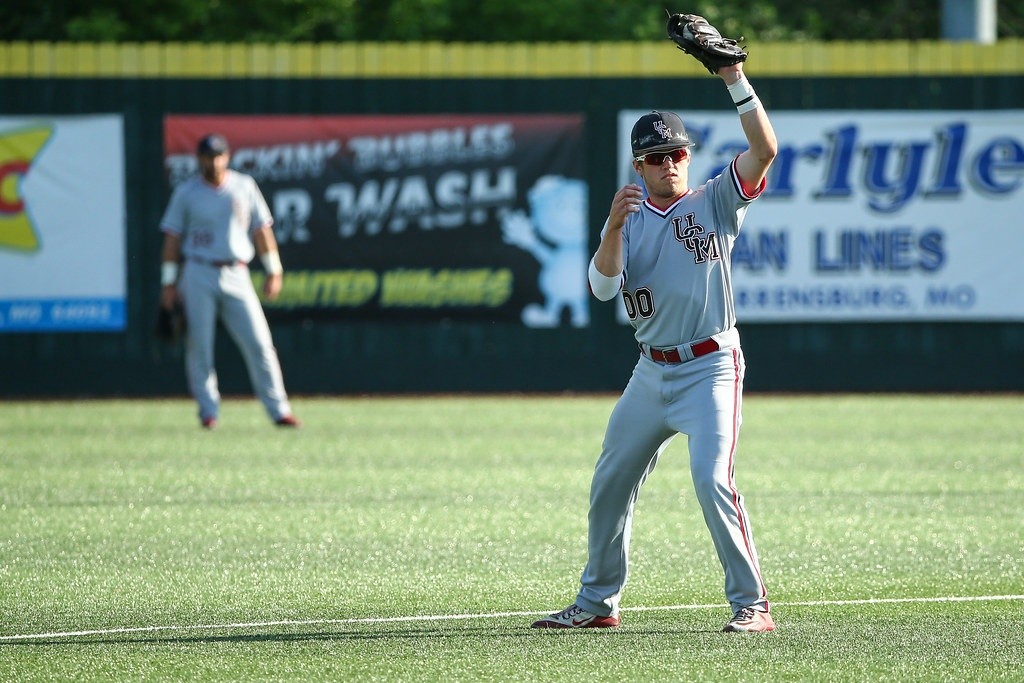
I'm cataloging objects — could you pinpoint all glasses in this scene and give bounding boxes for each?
[640,148,687,165]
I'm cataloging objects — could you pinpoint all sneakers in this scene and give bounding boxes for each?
[532,604,620,629]
[722,606,775,632]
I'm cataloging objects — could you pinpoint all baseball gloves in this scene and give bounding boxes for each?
[668,13,747,75]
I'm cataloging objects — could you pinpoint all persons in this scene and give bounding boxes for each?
[530,8,778,632]
[160,134,301,429]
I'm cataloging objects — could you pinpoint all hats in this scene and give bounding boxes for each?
[631,110,695,157]
[198,133,226,156]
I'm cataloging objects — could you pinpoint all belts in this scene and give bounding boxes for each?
[641,340,719,365]
[191,260,244,268]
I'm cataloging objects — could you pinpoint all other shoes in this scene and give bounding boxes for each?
[203,419,216,431]
[276,415,300,426]
